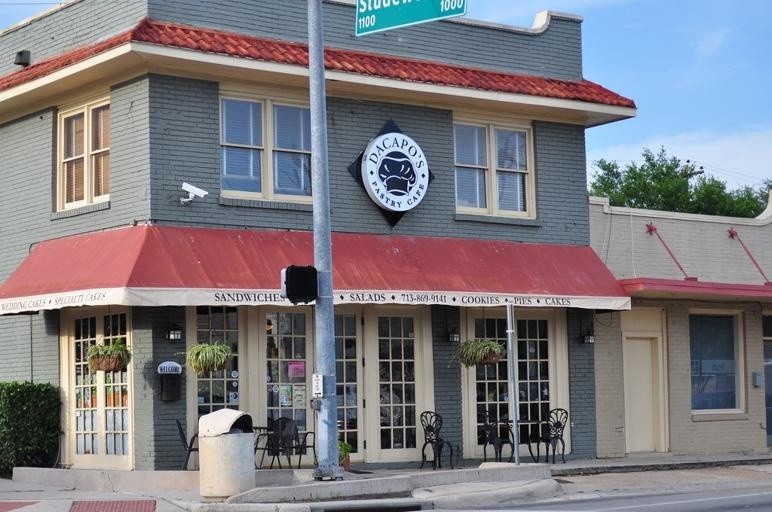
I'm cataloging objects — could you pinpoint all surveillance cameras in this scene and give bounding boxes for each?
[181,181,209,199]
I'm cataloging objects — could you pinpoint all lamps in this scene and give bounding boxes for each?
[165,320,182,341]
[584,328,594,344]
[448,328,459,342]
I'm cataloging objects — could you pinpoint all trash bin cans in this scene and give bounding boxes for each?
[199,409,256,498]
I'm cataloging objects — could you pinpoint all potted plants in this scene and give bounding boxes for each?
[86,338,131,372]
[338,439,352,471]
[454,338,505,368]
[187,342,232,374]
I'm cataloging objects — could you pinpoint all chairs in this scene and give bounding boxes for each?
[420,411,454,470]
[484,410,515,462]
[534,408,568,463]
[175,417,319,471]
